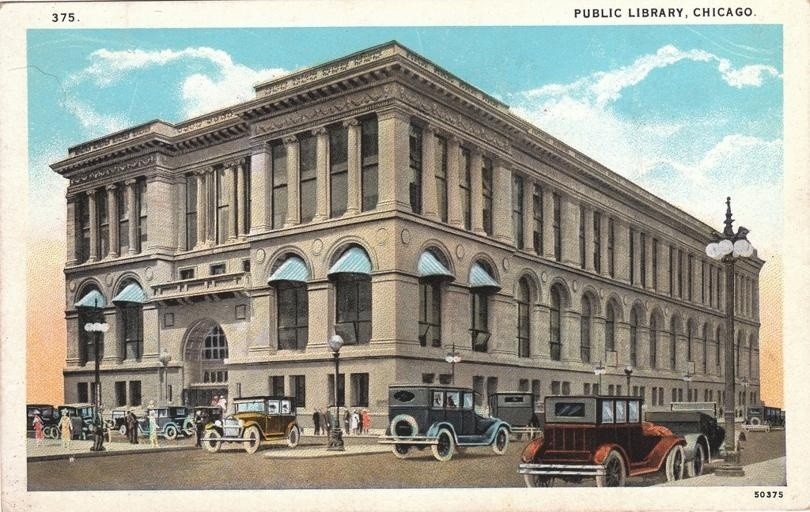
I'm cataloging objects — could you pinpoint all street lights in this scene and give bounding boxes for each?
[702,196,754,464]
[624,365,634,396]
[83,298,110,414]
[327,334,345,427]
[444,341,462,384]
[592,359,607,394]
[158,350,172,401]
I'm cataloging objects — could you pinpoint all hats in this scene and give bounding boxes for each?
[61,408,69,413]
[33,410,40,414]
[362,409,369,412]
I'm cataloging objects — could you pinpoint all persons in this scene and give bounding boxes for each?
[218,395,227,411]
[149,409,160,447]
[123,410,139,444]
[90,405,106,450]
[56,408,73,452]
[210,395,219,406]
[311,404,370,434]
[33,410,45,448]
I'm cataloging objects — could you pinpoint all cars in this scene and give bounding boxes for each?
[511,391,725,487]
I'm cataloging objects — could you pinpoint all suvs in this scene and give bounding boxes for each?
[376,382,545,462]
[27,394,302,454]
[745,405,785,434]
